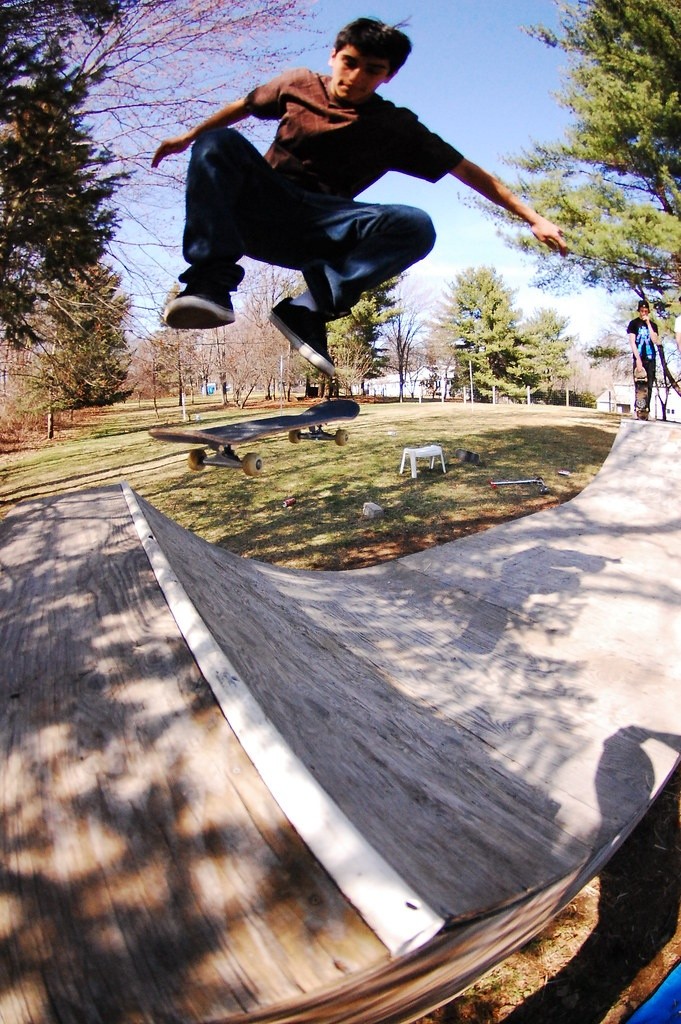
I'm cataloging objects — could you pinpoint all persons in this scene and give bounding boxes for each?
[674,295,681,352]
[151,18,571,376]
[627,300,659,419]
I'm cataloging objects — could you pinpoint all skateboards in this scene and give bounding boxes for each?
[454,448,480,464]
[148,399,361,476]
[634,367,651,419]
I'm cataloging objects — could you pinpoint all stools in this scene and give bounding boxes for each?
[400,445,446,479]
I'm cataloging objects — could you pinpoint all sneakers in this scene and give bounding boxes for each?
[164,283,235,329]
[269,297,336,377]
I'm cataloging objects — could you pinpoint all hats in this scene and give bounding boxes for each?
[637,301,649,311]
